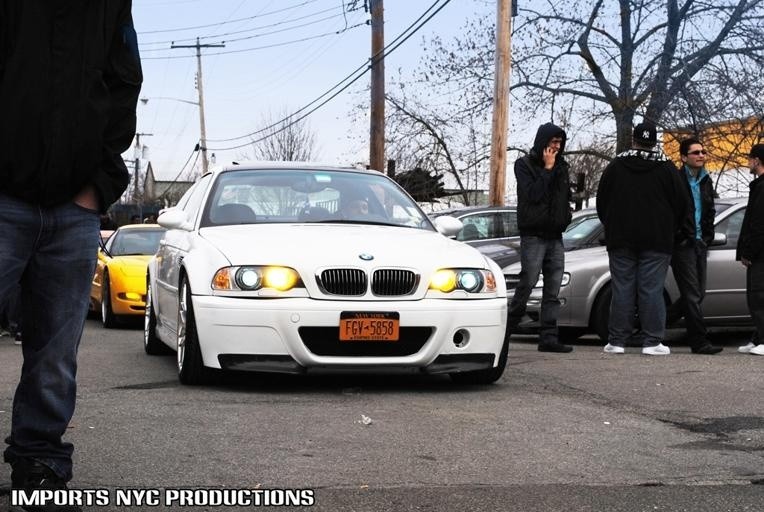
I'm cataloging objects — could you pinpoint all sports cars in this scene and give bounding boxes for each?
[88,223,171,329]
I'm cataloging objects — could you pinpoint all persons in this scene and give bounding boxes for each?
[595,122,684,355]
[463,224,479,240]
[668,138,725,355]
[0,1,140,510]
[506,122,578,354]
[336,188,372,221]
[735,144,763,354]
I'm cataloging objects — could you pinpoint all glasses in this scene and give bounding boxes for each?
[688,150,707,156]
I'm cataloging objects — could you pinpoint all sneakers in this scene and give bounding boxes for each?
[10,460,83,512]
[603,343,625,354]
[642,342,671,355]
[738,342,764,355]
[538,345,573,353]
[691,345,723,354]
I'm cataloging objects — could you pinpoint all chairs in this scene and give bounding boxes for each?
[214,204,257,225]
[299,206,329,220]
[456,224,478,240]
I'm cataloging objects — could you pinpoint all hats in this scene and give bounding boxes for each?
[634,123,657,146]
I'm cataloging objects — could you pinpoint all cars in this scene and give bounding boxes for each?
[140,160,513,392]
[421,205,606,270]
[501,196,759,349]
[98,230,115,251]
